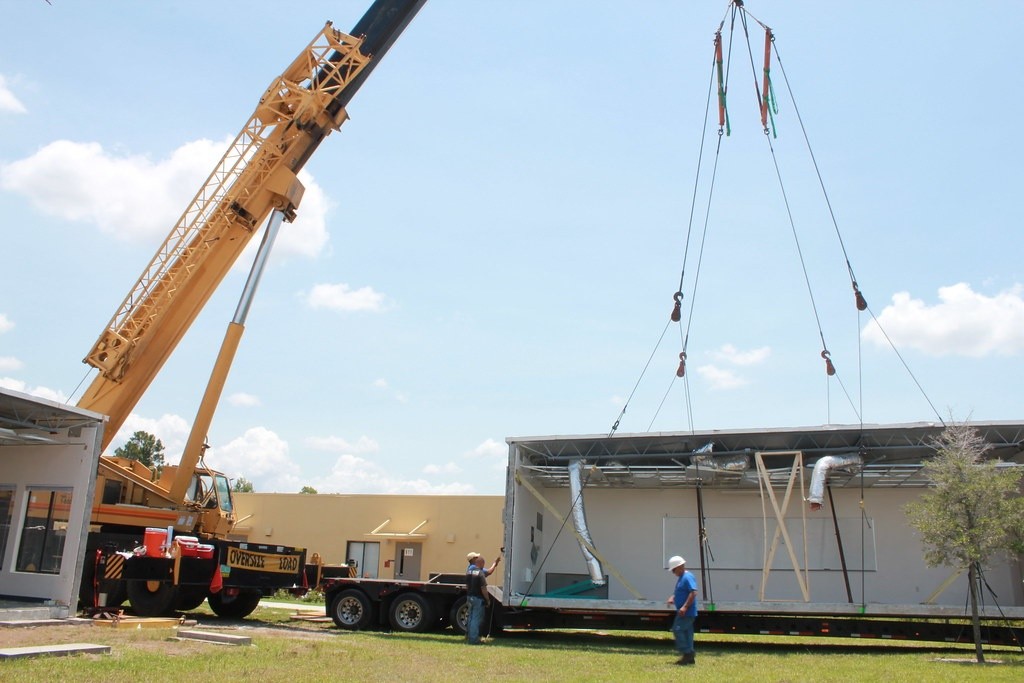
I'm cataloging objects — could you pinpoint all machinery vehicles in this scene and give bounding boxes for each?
[1,0,427,621]
[320,530,495,634]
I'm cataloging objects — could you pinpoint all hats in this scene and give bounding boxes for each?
[467,552,480,561]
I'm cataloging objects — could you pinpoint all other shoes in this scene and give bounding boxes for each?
[475,641,485,645]
[675,651,695,664]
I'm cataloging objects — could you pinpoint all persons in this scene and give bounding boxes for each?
[466,552,502,645]
[667,556,698,665]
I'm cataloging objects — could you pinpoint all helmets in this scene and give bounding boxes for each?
[669,555,686,571]
